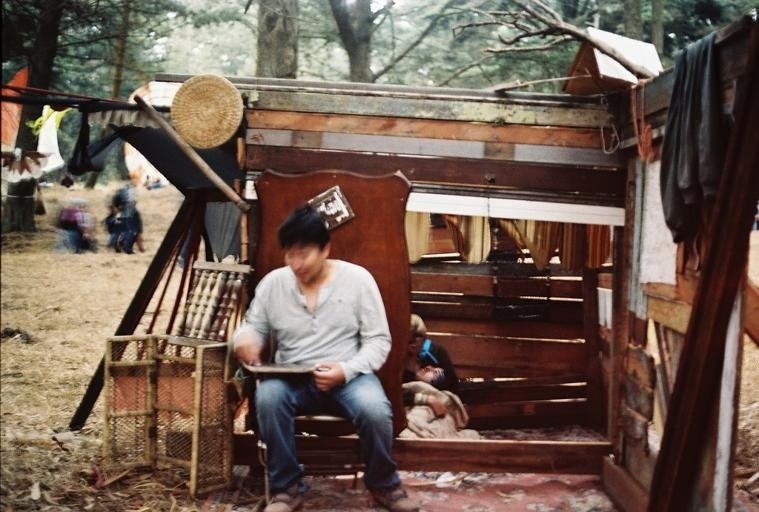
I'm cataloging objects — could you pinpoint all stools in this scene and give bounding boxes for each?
[248,410,363,492]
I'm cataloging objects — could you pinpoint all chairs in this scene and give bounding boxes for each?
[103,254,253,498]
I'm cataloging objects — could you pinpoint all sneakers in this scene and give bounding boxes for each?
[263,482,303,512]
[373,486,419,512]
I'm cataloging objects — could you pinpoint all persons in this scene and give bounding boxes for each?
[54,176,145,256]
[402,315,484,440]
[230,202,418,511]
